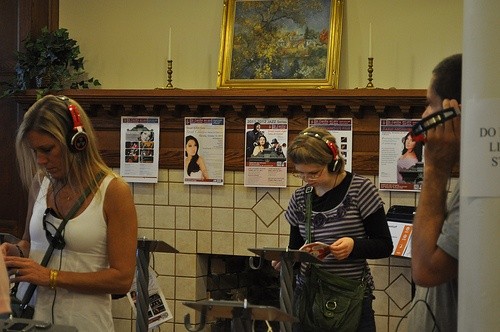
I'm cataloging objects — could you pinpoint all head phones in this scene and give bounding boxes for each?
[302,132,344,174]
[55,95,88,153]
[410,106,461,142]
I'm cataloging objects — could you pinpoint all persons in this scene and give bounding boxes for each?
[272,126,394,332]
[1,94,138,332]
[412,54,462,332]
[397,132,423,184]
[184,136,209,179]
[247,122,285,162]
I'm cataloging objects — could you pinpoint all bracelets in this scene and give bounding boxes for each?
[49,268,58,291]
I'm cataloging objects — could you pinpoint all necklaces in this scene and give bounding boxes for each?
[63,188,70,201]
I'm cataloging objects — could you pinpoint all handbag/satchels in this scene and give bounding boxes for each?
[293,263,368,332]
[8,290,36,332]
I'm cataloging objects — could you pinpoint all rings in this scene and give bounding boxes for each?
[15,269,21,275]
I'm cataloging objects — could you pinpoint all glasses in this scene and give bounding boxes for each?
[292,164,325,181]
